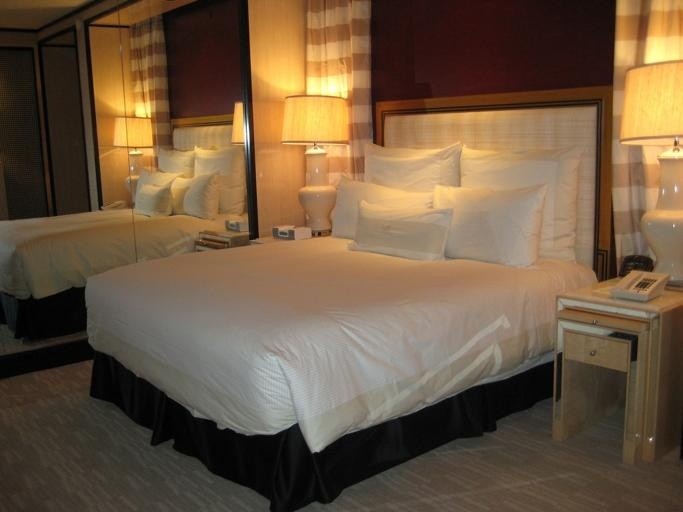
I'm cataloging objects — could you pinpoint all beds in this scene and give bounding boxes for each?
[81,81,616,511]
[0,113,248,348]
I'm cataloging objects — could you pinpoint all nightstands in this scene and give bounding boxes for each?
[251,235,326,247]
[191,232,248,252]
[551,276,683,468]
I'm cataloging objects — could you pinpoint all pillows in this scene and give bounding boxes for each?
[435,180,549,272]
[167,168,221,223]
[346,199,454,264]
[459,142,586,265]
[361,142,459,194]
[134,169,183,212]
[132,182,174,219]
[193,146,247,217]
[156,145,197,177]
[329,173,434,243]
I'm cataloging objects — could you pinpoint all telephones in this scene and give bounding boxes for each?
[609,270,670,302]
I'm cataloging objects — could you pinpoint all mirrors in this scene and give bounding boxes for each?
[0,0,261,380]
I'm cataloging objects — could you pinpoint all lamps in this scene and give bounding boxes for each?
[113,117,155,206]
[230,100,249,217]
[279,95,353,240]
[619,59,683,287]
